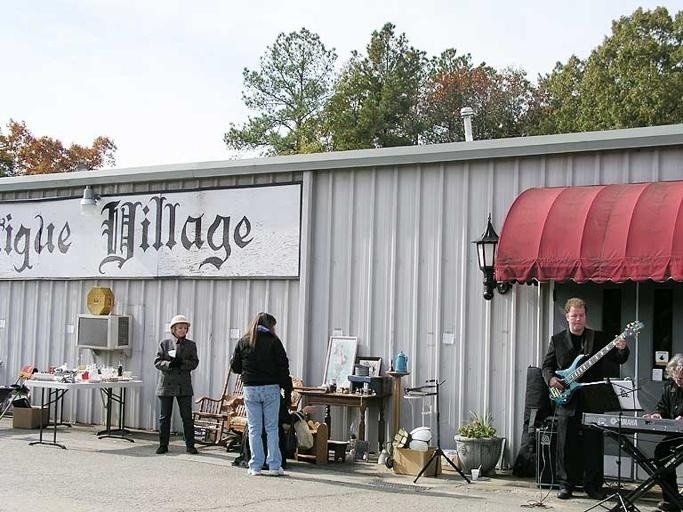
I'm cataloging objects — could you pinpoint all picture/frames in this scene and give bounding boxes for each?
[355,356,382,377]
[321,335,358,393]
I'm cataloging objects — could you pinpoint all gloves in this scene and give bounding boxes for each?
[169,360,182,369]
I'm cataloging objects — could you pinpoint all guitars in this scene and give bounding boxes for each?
[549,320,645,405]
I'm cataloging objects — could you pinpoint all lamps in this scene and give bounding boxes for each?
[76,183,102,219]
[468,210,538,301]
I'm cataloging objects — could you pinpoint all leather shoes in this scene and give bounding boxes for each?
[155,444,168,455]
[556,487,573,500]
[585,489,607,500]
[656,500,682,511]
[186,445,199,454]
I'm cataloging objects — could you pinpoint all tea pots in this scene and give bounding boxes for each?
[394,350,409,371]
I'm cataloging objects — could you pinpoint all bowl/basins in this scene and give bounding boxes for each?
[355,366,369,376]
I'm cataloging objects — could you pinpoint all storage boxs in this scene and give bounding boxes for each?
[11,406,48,430]
[392,444,442,477]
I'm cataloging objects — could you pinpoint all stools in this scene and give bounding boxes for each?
[326,439,350,465]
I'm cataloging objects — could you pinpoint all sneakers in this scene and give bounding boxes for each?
[247,466,262,477]
[269,467,285,476]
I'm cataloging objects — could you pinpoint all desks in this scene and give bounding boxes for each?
[299,389,392,442]
[22,378,143,451]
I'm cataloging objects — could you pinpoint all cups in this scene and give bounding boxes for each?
[470,469,479,482]
[49,362,139,382]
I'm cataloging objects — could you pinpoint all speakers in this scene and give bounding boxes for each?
[534,427,605,489]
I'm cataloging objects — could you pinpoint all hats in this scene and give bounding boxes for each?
[169,314,190,330]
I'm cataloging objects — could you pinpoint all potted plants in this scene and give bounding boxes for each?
[452,406,504,476]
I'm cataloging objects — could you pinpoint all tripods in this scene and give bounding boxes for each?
[585,411,649,511]
[413,379,472,486]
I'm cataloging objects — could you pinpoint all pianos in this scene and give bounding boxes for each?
[581,411,683,432]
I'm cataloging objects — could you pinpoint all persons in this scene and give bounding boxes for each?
[155,314,199,454]
[231,312,289,476]
[540,297,631,500]
[232,373,293,468]
[645,354,683,511]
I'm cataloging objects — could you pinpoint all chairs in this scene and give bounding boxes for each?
[0,368,38,422]
[191,362,243,447]
[223,373,304,453]
